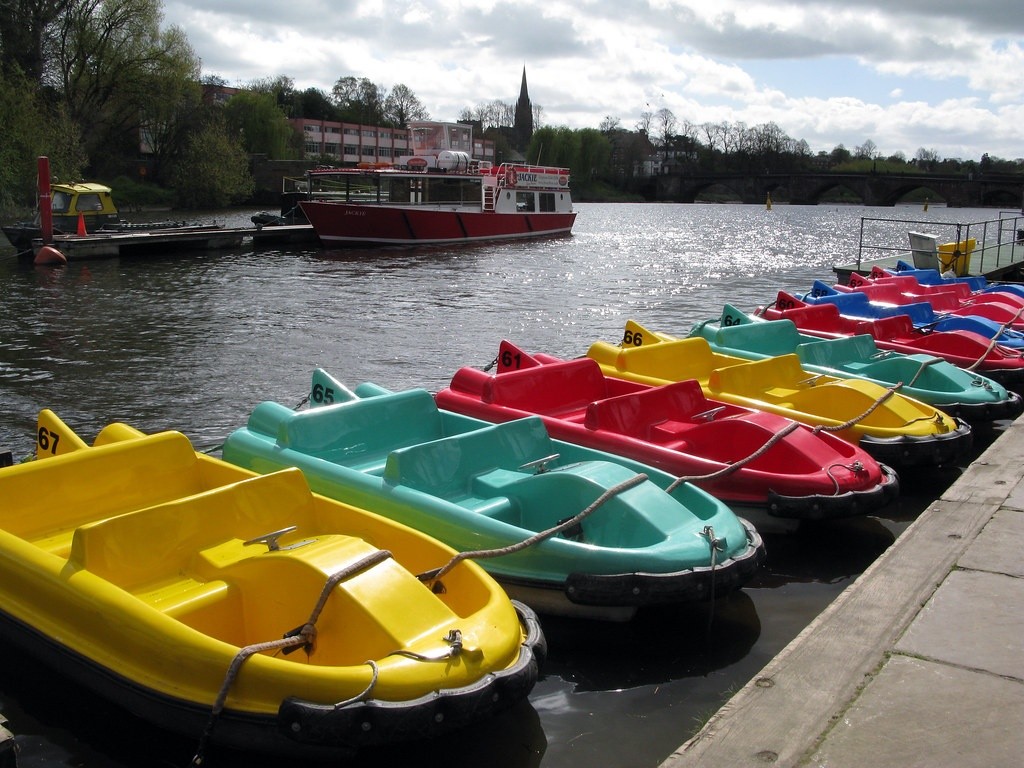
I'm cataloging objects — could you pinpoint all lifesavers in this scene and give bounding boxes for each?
[506,166,518,184]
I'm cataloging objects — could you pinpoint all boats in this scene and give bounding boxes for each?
[687,304,1024,422]
[223,368,767,622]
[1,176,225,249]
[435,339,900,525]
[0,409,549,758]
[297,150,577,249]
[586,321,973,466]
[754,260,1024,372]
